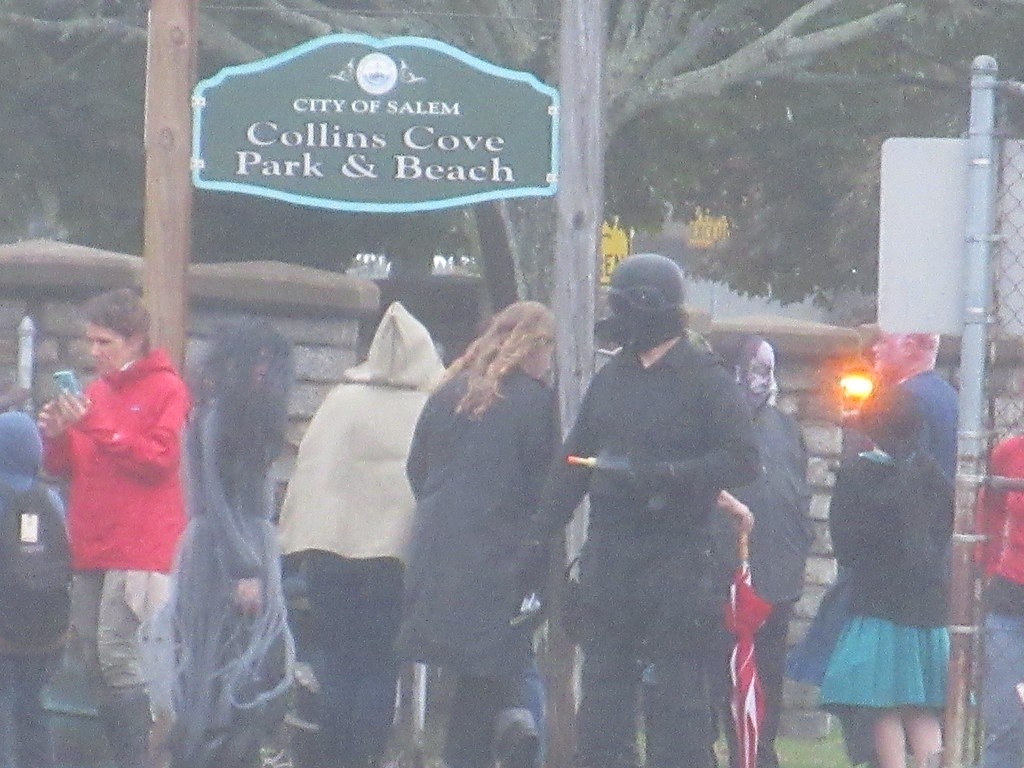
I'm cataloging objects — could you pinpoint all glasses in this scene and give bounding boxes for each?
[608,287,687,318]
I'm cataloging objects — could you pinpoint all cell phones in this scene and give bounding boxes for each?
[53,372,78,402]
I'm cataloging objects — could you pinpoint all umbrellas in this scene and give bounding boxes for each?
[721,519,776,768]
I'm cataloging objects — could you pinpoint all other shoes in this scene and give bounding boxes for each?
[496,710,541,768]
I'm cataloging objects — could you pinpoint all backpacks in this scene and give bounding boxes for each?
[1,478,73,643]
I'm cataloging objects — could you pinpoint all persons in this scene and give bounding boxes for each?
[0,411,73,768]
[136,320,295,768]
[403,301,564,768]
[272,300,447,768]
[694,336,810,768]
[780,325,959,768]
[817,386,958,768]
[970,430,1023,768]
[494,253,760,768]
[36,289,193,768]
